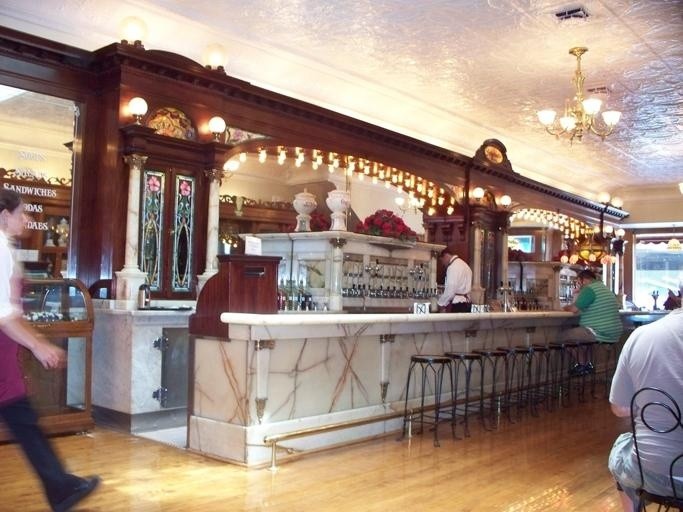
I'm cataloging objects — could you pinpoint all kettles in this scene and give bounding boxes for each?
[137,284,151,311]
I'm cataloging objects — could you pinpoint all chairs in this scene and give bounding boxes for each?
[617,386,683,511]
[396,341,614,446]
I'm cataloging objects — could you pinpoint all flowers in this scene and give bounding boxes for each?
[356,208,415,241]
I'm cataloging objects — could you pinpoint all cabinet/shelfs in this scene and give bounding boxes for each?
[22,204,71,279]
[22,278,96,435]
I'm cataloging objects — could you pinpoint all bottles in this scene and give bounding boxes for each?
[277,279,312,311]
[512,290,527,312]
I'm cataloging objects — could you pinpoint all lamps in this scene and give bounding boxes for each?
[560,191,625,264]
[665,225,680,250]
[500,195,510,209]
[535,45,621,143]
[127,97,147,124]
[207,116,227,143]
[474,186,484,204]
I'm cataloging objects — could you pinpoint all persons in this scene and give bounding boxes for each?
[437,248,472,311]
[554,269,623,377]
[666,283,682,307]
[0,188,99,512]
[608,279,682,511]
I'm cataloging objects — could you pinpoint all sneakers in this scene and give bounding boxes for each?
[567,363,593,375]
[52,475,98,511]
